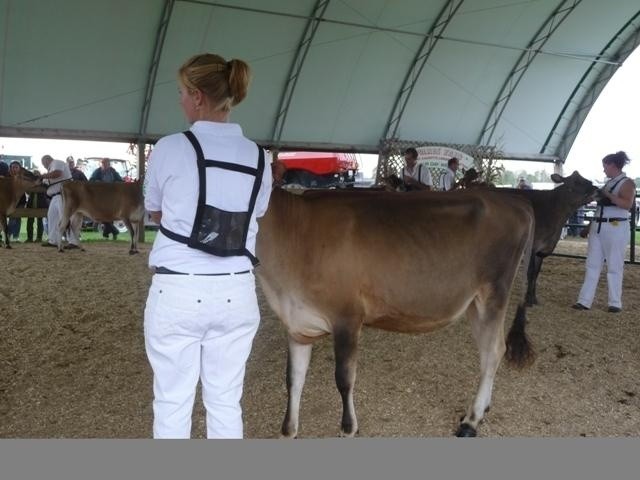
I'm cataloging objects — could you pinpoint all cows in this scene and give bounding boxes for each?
[496,170,601,308]
[56,174,145,255]
[0,165,48,250]
[252,184,536,439]
[272,166,484,191]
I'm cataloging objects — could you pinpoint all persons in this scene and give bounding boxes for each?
[142,53,274,440]
[0,155,124,250]
[438,157,460,191]
[570,151,636,312]
[515,177,534,188]
[396,147,433,192]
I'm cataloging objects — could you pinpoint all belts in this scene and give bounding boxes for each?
[51,190,61,198]
[595,218,627,222]
[155,266,250,275]
